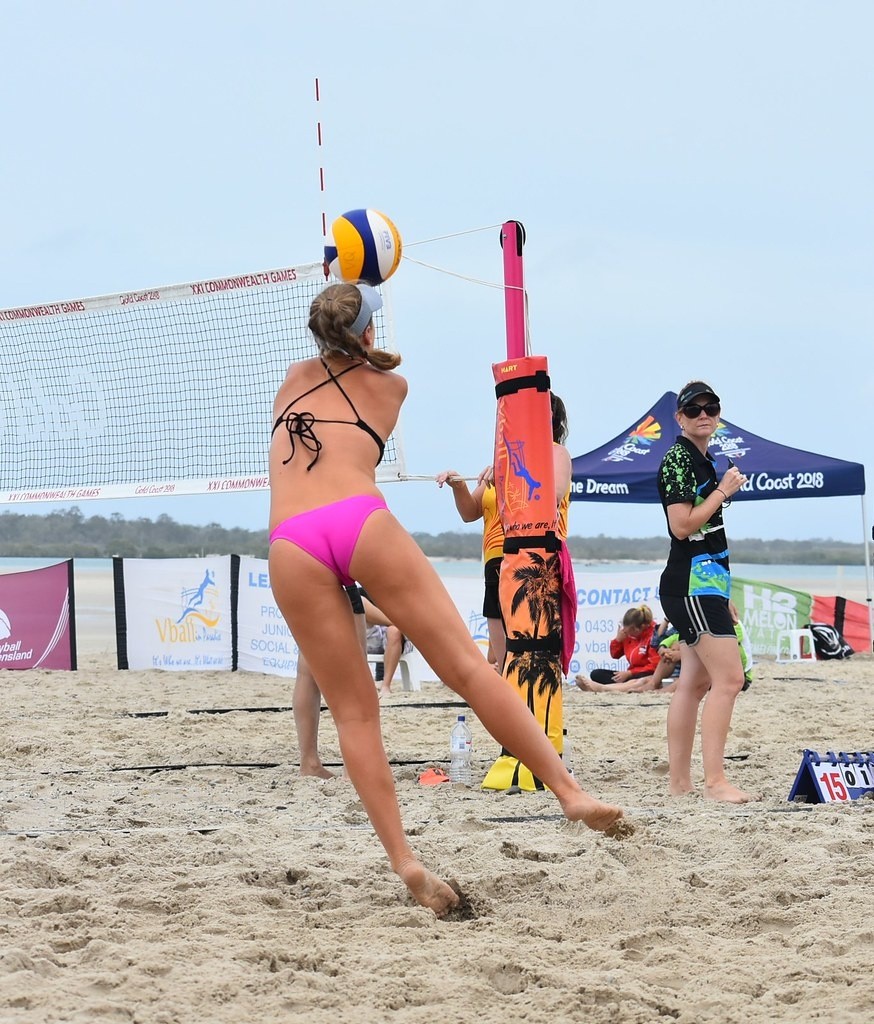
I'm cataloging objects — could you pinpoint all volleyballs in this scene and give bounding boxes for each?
[322,207,403,287]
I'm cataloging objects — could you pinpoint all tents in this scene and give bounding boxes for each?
[569,392,874,655]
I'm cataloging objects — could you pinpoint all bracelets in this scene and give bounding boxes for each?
[713,486,729,501]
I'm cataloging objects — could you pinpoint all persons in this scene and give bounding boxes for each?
[267,281,625,918]
[436,390,579,678]
[574,381,764,806]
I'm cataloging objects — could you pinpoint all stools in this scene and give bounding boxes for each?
[776,628,817,665]
[365,653,421,691]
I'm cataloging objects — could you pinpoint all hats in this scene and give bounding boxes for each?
[347,284,383,341]
[677,383,720,407]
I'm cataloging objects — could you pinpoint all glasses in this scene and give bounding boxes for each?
[682,403,720,419]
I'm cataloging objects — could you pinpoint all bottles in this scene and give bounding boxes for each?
[449,715,472,784]
